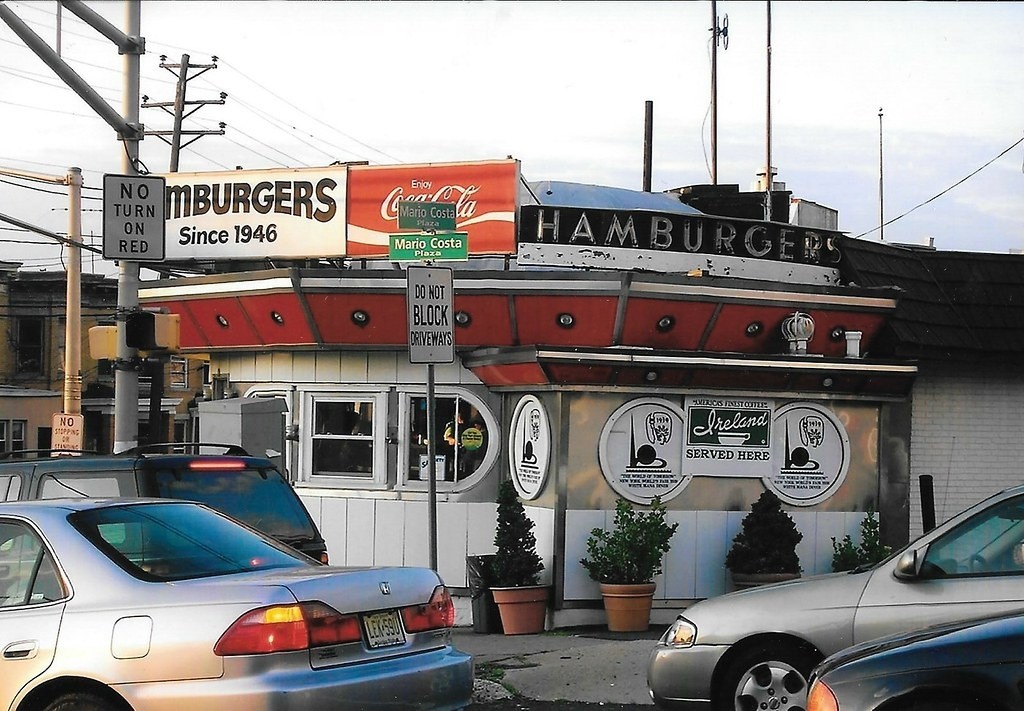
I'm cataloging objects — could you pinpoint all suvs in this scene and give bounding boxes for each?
[0,442,330,574]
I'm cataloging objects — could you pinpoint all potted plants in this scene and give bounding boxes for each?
[724,489,804,589]
[579,495,679,631]
[488,480,551,635]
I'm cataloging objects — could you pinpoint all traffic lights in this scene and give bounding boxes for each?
[125,311,180,355]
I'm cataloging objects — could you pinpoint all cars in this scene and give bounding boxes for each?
[645,483,1024,711]
[0,498,476,711]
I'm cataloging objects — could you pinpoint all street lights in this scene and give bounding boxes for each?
[877,107,885,242]
